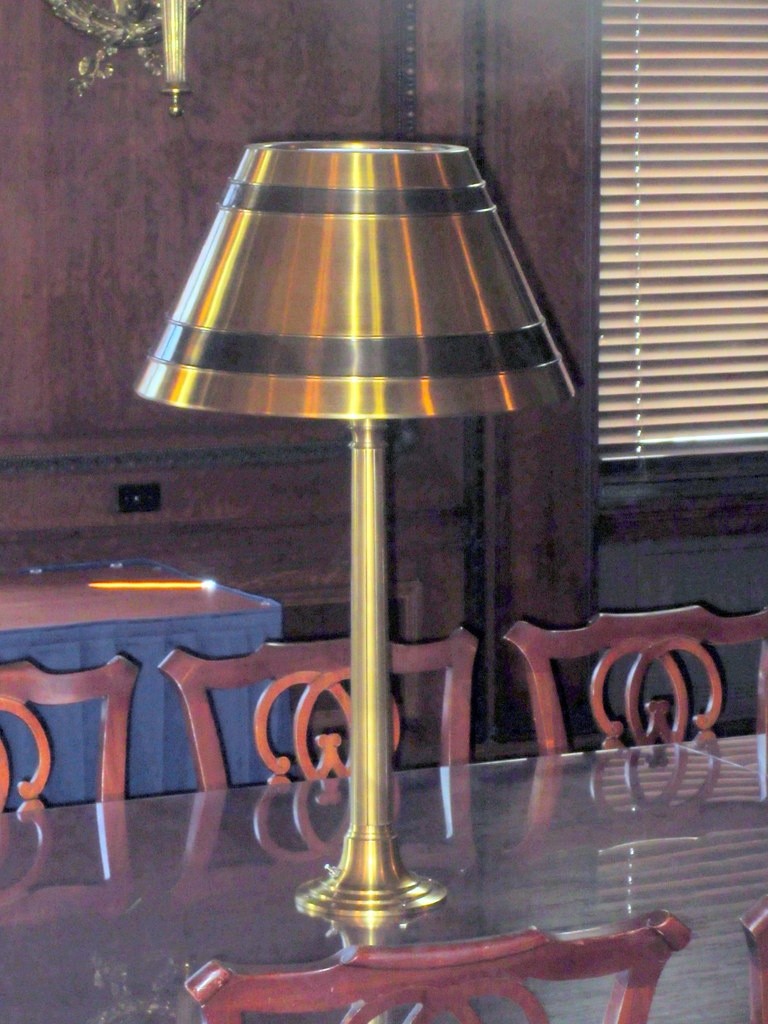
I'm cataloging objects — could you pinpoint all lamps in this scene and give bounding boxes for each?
[131,139,578,1024]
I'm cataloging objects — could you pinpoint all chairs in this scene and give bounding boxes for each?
[156,625,479,793]
[502,602,768,757]
[0,655,140,813]
[183,906,693,1024]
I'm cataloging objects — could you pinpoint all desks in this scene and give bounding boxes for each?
[0,555,293,813]
[0,733,768,1024]
